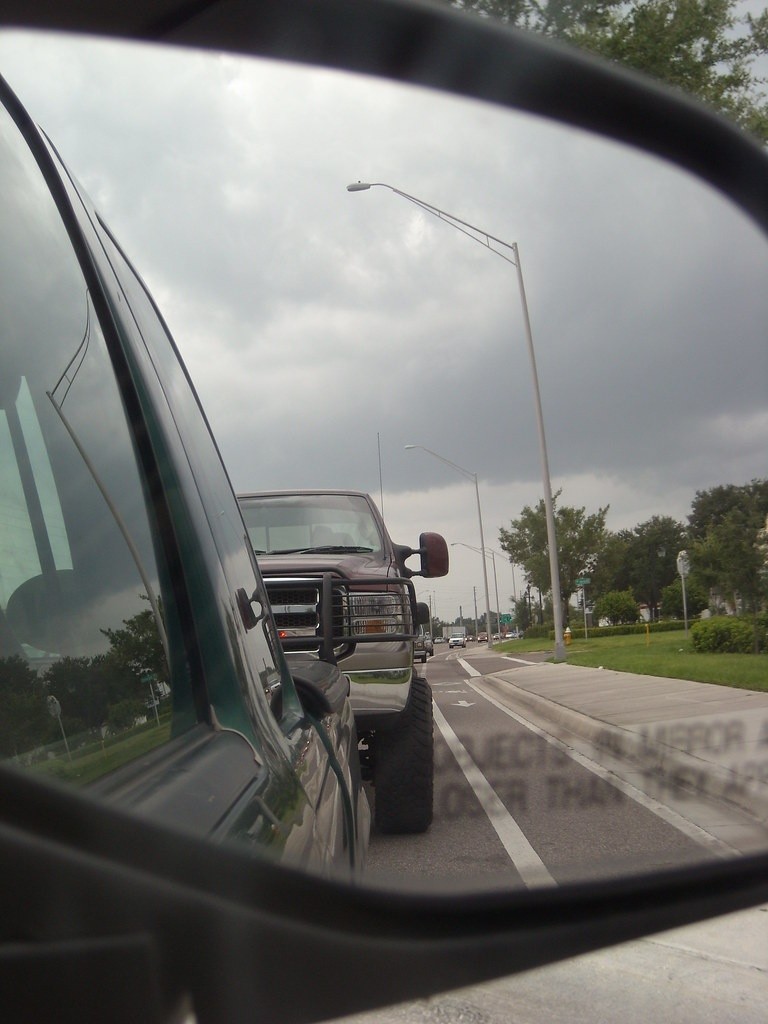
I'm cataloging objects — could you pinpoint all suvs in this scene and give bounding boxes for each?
[449,633,467,649]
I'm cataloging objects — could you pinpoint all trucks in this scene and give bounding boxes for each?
[240,491,448,837]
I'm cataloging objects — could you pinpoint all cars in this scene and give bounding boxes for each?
[506,632,517,638]
[414,624,437,663]
[493,633,504,640]
[0,71,385,897]
[434,637,445,644]
[466,635,475,642]
[478,632,488,643]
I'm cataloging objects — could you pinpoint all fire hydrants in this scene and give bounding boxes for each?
[564,626,572,645]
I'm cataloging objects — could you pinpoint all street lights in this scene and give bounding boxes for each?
[402,444,493,647]
[345,180,566,663]
[450,543,502,644]
[479,546,520,639]
